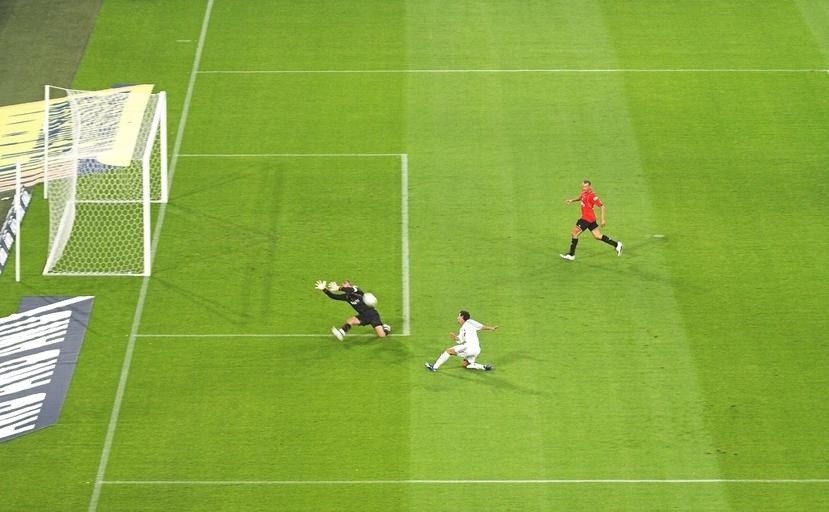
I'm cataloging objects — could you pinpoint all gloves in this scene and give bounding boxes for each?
[328,282,340,291]
[315,281,326,290]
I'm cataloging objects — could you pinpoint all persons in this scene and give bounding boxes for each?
[557,181,625,261]
[423,310,499,373]
[313,278,392,340]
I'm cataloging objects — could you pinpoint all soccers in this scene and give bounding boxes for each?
[363,294,376,307]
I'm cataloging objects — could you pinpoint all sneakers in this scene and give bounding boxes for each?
[561,254,574,260]
[426,363,438,372]
[615,242,623,256]
[484,365,493,371]
[332,327,345,342]
[384,324,392,332]
